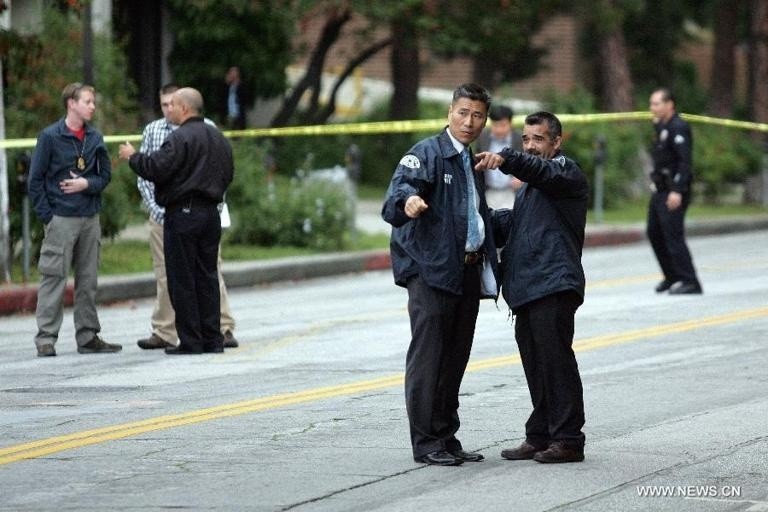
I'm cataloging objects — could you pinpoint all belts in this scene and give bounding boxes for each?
[465,252,484,265]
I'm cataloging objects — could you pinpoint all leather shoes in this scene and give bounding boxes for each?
[534,440,585,463]
[501,441,537,460]
[416,450,483,465]
[656,279,702,294]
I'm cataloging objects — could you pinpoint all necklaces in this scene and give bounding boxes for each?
[71,131,88,171]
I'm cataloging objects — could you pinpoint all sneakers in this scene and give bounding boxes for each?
[137,331,238,354]
[77,335,121,353]
[37,344,56,356]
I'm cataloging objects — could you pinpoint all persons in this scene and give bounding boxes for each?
[218,64,247,128]
[26,81,126,359]
[472,110,592,465]
[379,82,503,467]
[642,88,705,296]
[133,82,239,352]
[114,85,236,356]
[476,103,524,209]
